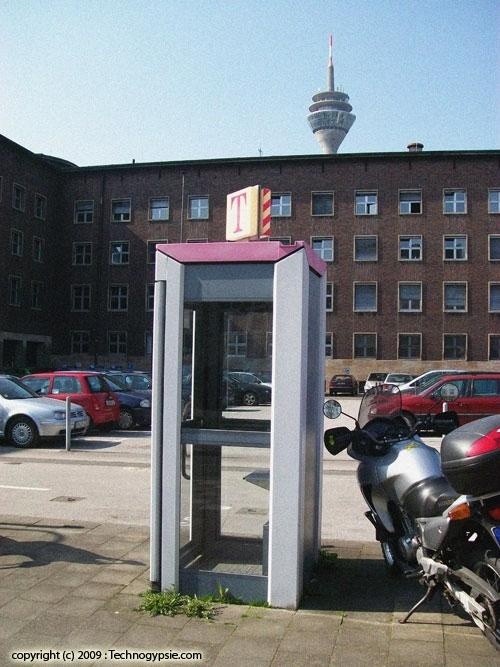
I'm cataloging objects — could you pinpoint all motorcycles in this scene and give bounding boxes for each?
[317,382,500,652]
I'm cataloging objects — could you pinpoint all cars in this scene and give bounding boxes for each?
[330,370,500,434]
[0,363,271,448]
